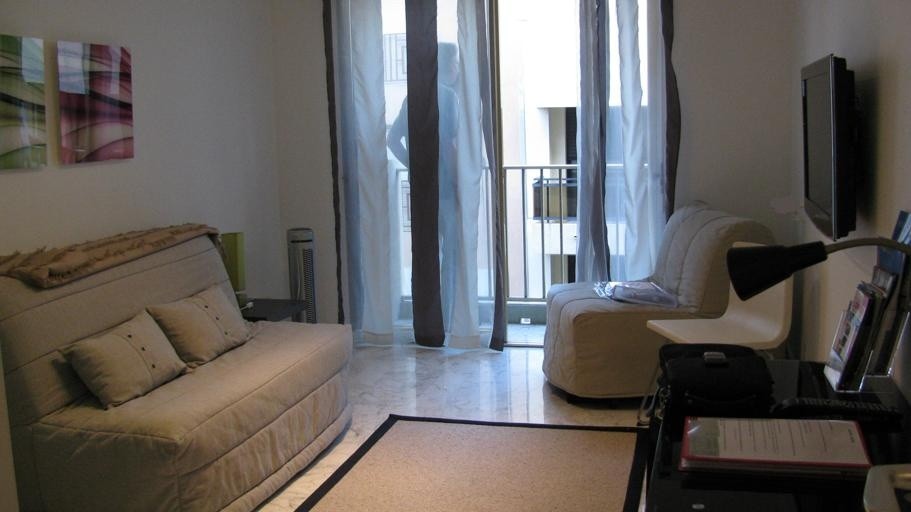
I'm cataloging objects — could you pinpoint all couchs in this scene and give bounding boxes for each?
[0,220,353,512]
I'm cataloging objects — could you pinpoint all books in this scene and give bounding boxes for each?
[824,210,911,393]
[679,417,872,478]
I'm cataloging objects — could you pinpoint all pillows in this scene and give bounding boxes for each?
[54,285,255,412]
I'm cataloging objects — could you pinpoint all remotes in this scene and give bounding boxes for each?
[768,398,903,422]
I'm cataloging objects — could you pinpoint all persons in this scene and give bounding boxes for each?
[386,42,459,331]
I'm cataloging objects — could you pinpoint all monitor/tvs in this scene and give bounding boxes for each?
[801,53,859,242]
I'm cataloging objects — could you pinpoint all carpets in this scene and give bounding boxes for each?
[294,414,649,511]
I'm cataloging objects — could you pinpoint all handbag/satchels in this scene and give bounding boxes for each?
[655,343,779,414]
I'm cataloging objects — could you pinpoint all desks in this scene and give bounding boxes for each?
[240,297,310,324]
[645,358,910,512]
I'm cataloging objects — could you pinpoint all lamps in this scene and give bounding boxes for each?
[725,236,909,302]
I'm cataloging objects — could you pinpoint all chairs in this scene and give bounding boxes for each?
[542,201,789,399]
[643,238,793,413]
